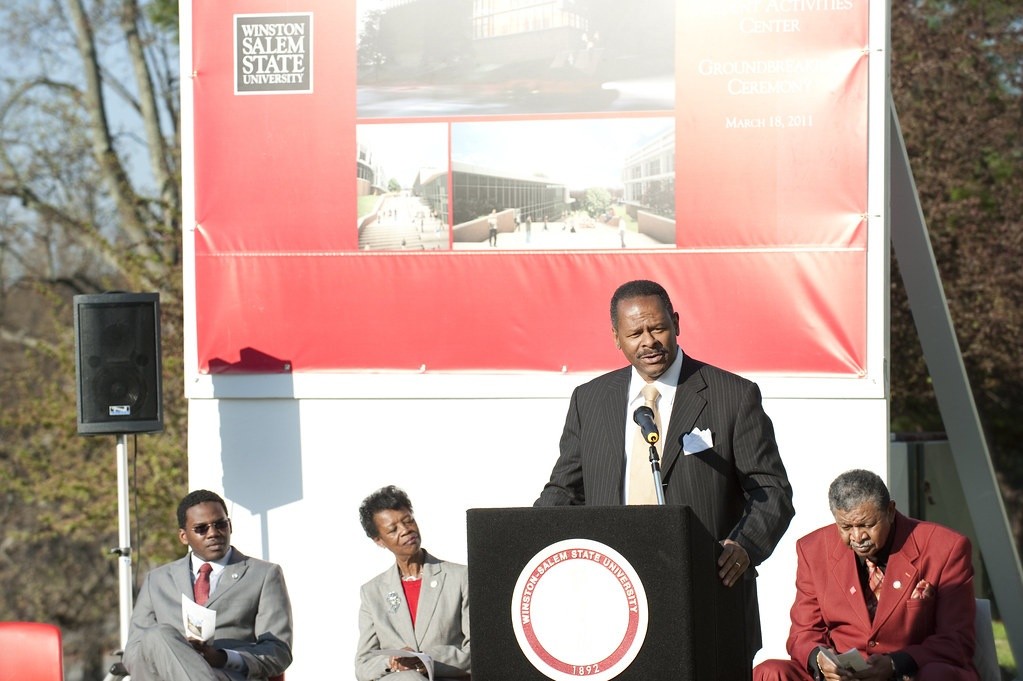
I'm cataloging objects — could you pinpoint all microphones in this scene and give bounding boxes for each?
[633,406,660,443]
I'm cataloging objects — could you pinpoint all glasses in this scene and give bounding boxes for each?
[186,519,230,535]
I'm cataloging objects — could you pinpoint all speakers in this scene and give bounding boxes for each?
[73,291,165,436]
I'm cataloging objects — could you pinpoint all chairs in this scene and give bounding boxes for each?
[973,597,1004,681]
[0,621,63,681]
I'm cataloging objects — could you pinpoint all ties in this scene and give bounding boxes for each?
[627,384,663,505]
[865,556,884,622]
[193,562,214,606]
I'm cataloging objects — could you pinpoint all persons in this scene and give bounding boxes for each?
[354,485,472,681]
[617,216,626,248]
[122,489,293,681]
[753,470,982,681]
[514,212,520,232]
[570,226,576,232]
[488,208,497,247]
[544,216,547,230]
[533,280,796,681]
[378,209,444,252]
[560,221,567,230]
[525,216,531,241]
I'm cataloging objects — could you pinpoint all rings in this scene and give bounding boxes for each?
[736,562,741,567]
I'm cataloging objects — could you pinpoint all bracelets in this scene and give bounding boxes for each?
[816,651,824,675]
[891,658,897,670]
